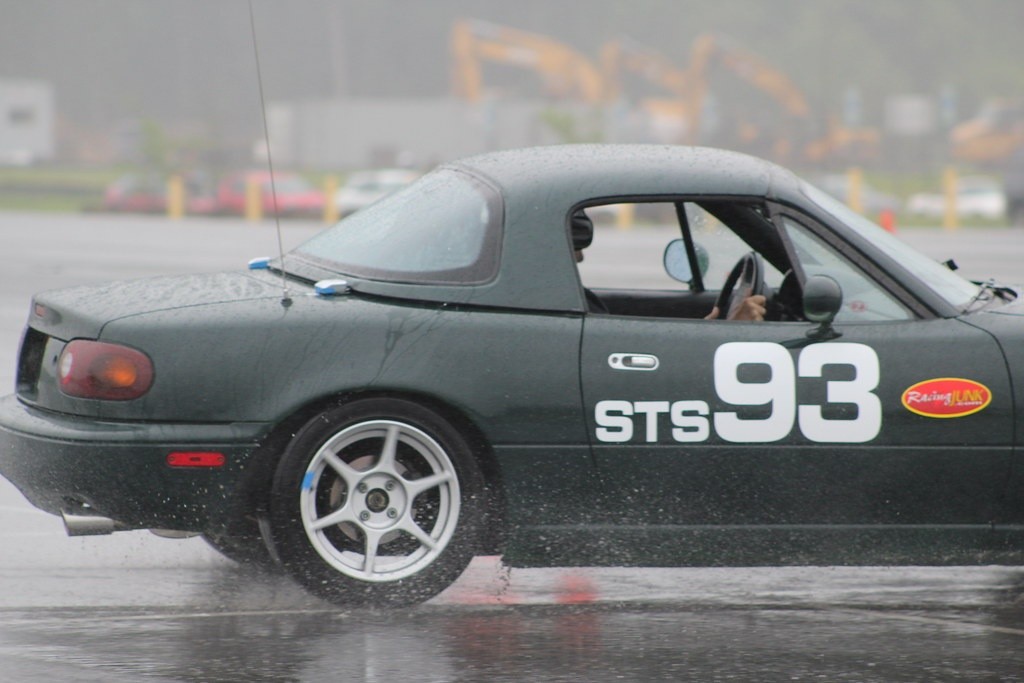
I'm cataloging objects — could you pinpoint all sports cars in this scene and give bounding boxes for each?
[1,141,1024,613]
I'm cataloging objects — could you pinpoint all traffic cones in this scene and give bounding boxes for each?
[879,207,895,234]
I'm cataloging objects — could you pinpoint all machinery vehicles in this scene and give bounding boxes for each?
[444,12,1023,209]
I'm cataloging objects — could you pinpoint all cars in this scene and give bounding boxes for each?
[102,161,424,218]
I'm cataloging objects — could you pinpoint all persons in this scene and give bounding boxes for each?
[572,209,766,324]
